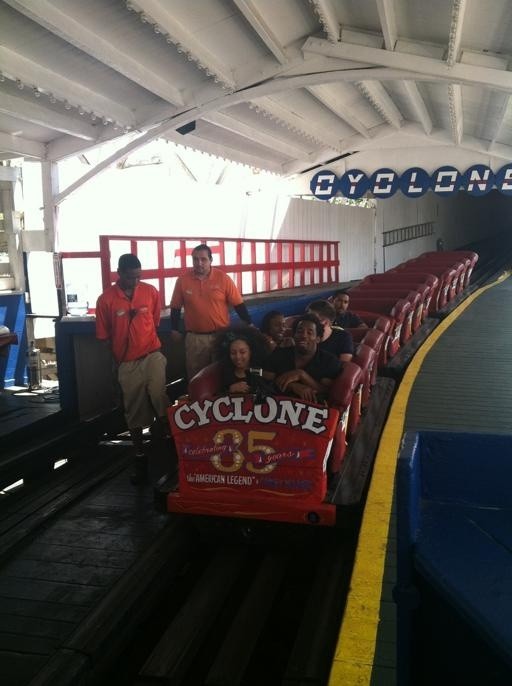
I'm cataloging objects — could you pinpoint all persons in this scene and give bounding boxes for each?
[170,245,254,380]
[95,253,178,474]
[218,290,368,409]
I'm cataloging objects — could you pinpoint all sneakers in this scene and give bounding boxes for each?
[131,455,149,485]
